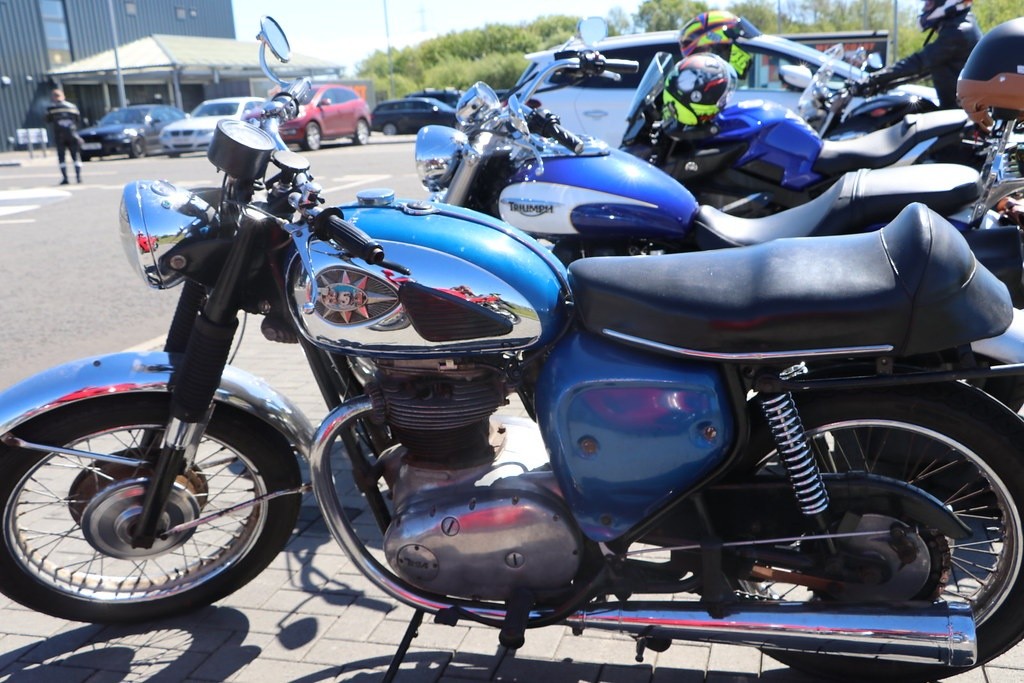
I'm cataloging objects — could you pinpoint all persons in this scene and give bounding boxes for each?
[856,0,985,110]
[43,89,81,185]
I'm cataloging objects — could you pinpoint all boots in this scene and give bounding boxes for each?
[75,166,82,183]
[60,167,69,185]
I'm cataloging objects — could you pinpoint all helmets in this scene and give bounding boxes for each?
[919,0,972,33]
[956,14,1024,132]
[663,52,737,131]
[677,9,760,80]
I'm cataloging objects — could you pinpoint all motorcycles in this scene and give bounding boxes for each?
[0,14,1024,683]
[415,14,1024,443]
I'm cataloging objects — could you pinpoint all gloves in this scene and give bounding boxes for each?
[845,79,864,96]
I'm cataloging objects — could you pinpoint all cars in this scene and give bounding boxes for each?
[368,98,465,136]
[498,25,945,152]
[239,79,376,149]
[159,96,269,159]
[80,103,189,163]
[403,87,466,111]
